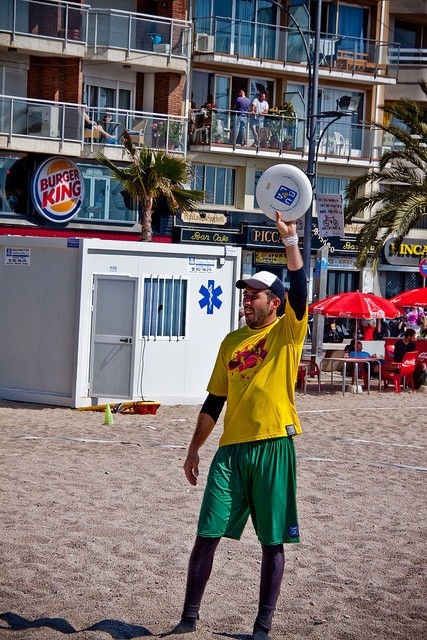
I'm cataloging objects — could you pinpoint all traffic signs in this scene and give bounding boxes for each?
[420,258,427,277]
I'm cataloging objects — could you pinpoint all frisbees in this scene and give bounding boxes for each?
[256,164,313,224]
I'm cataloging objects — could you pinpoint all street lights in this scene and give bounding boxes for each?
[267,97,363,281]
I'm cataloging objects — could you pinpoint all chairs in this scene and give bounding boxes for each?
[298,360,321,396]
[381,351,418,394]
[350,353,371,387]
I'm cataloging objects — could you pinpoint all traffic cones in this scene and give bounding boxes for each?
[103,403,114,425]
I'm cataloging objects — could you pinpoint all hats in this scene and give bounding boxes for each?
[235,270,286,304]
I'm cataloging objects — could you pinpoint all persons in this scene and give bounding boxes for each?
[393,329,427,363]
[251,92,269,147]
[200,101,214,144]
[80,102,117,140]
[232,89,251,145]
[159,208,308,640]
[350,339,402,374]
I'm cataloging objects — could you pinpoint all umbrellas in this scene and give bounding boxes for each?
[308,292,401,319]
[387,287,427,309]
